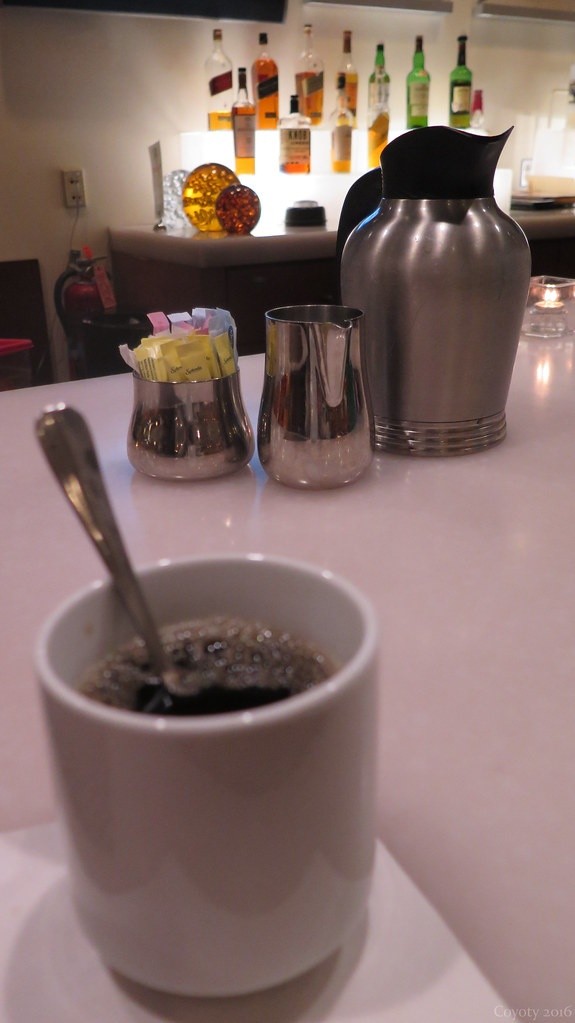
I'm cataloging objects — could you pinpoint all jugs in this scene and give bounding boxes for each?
[335,126,531,458]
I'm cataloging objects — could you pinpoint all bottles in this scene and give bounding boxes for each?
[208,29,232,129]
[368,45,389,107]
[330,71,351,174]
[449,36,471,128]
[295,25,323,125]
[232,68,256,175]
[252,33,278,129]
[366,89,389,168]
[406,35,430,129]
[335,31,358,117]
[472,89,487,136]
[281,95,310,173]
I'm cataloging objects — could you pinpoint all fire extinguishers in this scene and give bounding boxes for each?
[54,251,117,381]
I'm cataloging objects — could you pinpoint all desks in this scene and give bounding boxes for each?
[0,335,574,1023]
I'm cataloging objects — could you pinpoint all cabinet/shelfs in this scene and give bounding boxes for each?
[109,237,575,355]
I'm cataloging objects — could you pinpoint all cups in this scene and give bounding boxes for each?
[36,550,378,996]
[258,305,372,490]
[127,371,254,480]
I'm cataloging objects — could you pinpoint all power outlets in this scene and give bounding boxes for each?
[63,169,86,209]
[519,159,532,189]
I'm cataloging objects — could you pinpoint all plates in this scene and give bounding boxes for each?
[0,822,519,1023]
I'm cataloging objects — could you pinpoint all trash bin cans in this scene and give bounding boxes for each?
[81,311,152,376]
